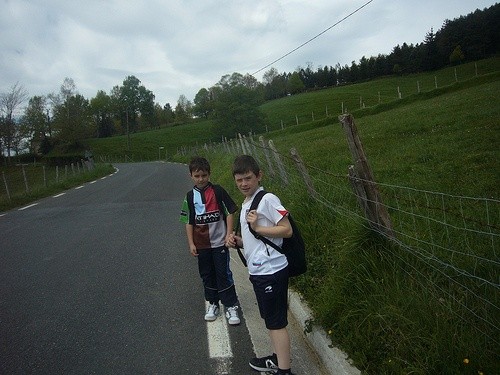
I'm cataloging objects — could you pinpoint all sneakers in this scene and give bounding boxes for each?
[225,306,241,325]
[204,305,220,321]
[249,355,292,375]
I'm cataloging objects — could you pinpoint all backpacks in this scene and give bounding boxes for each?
[233,191,309,278]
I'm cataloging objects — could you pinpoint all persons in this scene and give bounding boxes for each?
[226,156,295,375]
[179,156,240,324]
